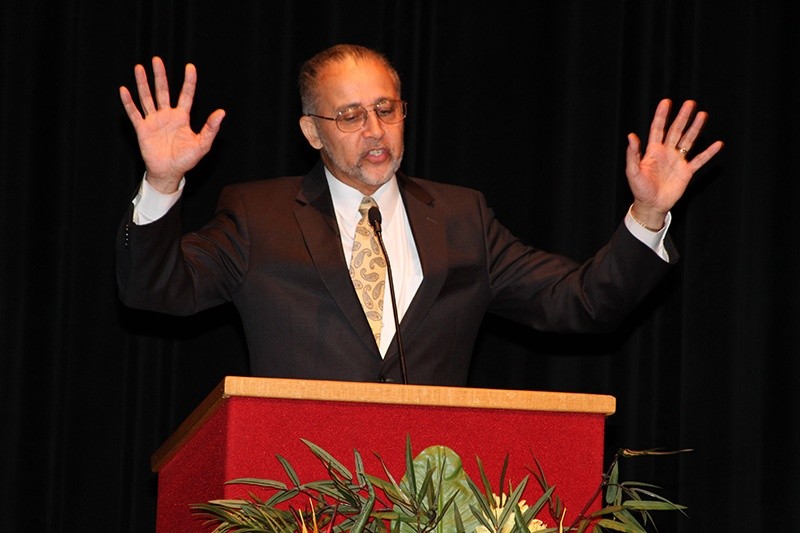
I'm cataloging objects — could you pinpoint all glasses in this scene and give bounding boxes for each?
[307,100,406,132]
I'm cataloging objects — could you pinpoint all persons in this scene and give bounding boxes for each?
[115,44,723,388]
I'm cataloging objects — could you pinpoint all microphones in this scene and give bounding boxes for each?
[367,205,411,384]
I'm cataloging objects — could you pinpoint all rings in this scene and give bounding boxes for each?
[677,147,687,155]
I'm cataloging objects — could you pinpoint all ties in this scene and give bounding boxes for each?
[349,196,385,347]
